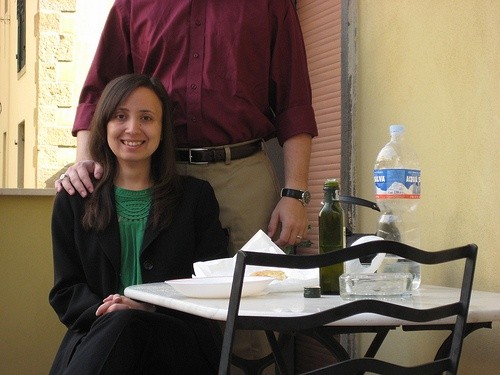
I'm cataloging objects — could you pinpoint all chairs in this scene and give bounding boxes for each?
[219,239,478,375]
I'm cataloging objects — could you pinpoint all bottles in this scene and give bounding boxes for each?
[373,123,424,292]
[317,178,346,296]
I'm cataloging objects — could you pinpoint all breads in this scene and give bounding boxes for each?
[252,270,286,280]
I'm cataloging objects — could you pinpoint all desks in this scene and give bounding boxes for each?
[124,278,500,375]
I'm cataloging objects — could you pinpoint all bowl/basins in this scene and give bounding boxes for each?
[164,275,274,298]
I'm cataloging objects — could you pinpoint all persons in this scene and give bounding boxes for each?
[47,72,231,375]
[54,0,318,375]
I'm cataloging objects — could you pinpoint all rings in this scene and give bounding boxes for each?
[59,174,68,179]
[297,234,303,239]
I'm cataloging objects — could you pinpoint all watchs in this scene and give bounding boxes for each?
[279,187,312,207]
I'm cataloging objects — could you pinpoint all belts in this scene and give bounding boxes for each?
[177,138,264,165]
